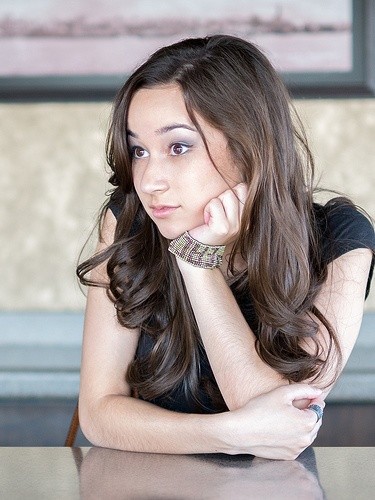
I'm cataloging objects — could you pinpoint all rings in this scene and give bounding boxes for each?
[307,403,323,422]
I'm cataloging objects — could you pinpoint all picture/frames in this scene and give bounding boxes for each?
[0,0,375,102]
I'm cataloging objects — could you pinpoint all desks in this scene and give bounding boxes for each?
[0,447,375,500]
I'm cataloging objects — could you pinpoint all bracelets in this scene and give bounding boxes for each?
[167,231,226,270]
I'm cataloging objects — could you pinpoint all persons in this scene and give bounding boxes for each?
[78,35,375,460]
